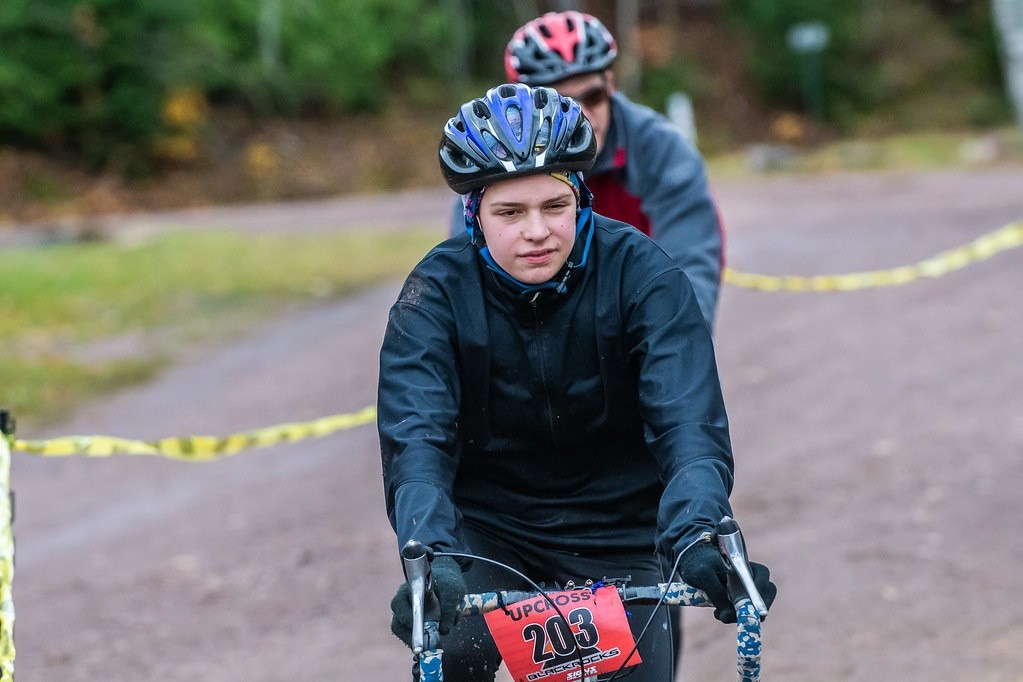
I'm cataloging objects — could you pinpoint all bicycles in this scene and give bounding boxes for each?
[401,513,770,682]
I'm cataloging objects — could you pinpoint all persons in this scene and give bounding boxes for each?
[377,83,777,682]
[453,10,726,343]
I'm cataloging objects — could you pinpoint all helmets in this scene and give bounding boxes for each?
[438,83,598,195]
[504,10,618,86]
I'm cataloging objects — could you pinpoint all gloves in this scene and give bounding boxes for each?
[671,542,778,624]
[390,555,465,650]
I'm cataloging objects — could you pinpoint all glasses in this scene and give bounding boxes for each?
[575,87,607,108]
